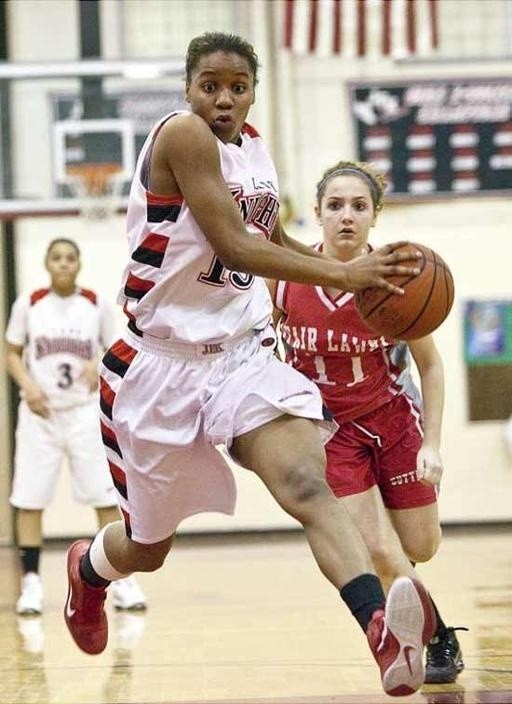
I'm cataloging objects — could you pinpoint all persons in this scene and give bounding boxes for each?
[64,32,436,697]
[264,162,465,686]
[3,238,147,616]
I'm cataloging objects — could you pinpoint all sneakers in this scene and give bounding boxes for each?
[16,571,44,615]
[65,539,148,653]
[364,574,469,697]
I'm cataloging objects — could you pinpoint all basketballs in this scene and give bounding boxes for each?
[354,241,453,341]
